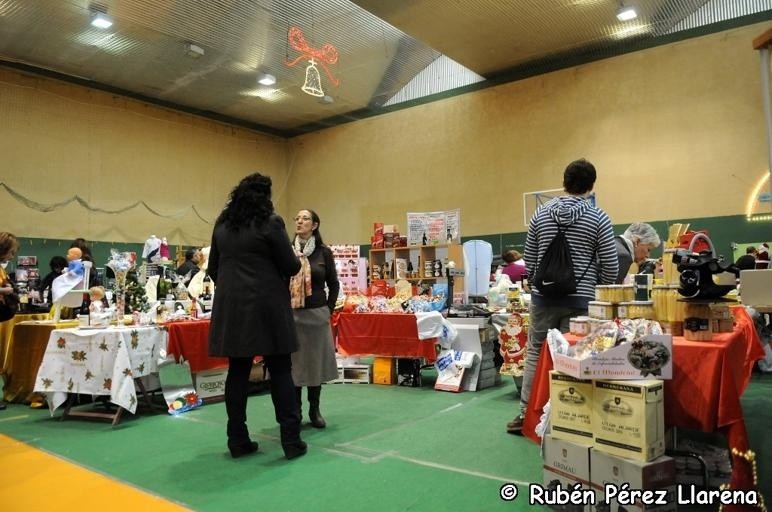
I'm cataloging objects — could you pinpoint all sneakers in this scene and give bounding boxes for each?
[281,441,308,459]
[507,415,524,431]
[227,441,258,457]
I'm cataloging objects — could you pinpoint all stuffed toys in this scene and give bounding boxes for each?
[54,247,83,303]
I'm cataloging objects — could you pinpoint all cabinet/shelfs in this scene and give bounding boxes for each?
[369,245,465,303]
[327,244,367,295]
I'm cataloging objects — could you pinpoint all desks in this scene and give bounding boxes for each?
[524,306,767,511]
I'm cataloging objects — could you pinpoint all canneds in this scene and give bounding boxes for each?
[575,319,589,337]
[569,318,577,335]
[587,284,656,320]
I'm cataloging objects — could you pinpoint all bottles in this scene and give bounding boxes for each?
[78,292,89,326]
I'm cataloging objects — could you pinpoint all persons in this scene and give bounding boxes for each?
[207,172,308,460]
[735,245,756,270]
[141,234,162,263]
[34,256,68,292]
[505,159,619,431]
[69,237,97,319]
[501,250,525,282]
[177,247,202,288]
[615,222,661,283]
[287,207,341,429]
[1,231,23,409]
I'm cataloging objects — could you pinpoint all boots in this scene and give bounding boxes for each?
[309,401,325,427]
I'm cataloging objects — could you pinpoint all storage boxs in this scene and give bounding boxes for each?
[537,366,679,512]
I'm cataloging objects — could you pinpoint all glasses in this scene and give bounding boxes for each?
[293,216,312,221]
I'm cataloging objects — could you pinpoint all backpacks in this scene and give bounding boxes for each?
[535,224,598,295]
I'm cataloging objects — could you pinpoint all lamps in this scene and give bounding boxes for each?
[256,71,278,86]
[616,4,639,24]
[89,10,114,29]
[319,94,332,105]
[183,41,205,60]
